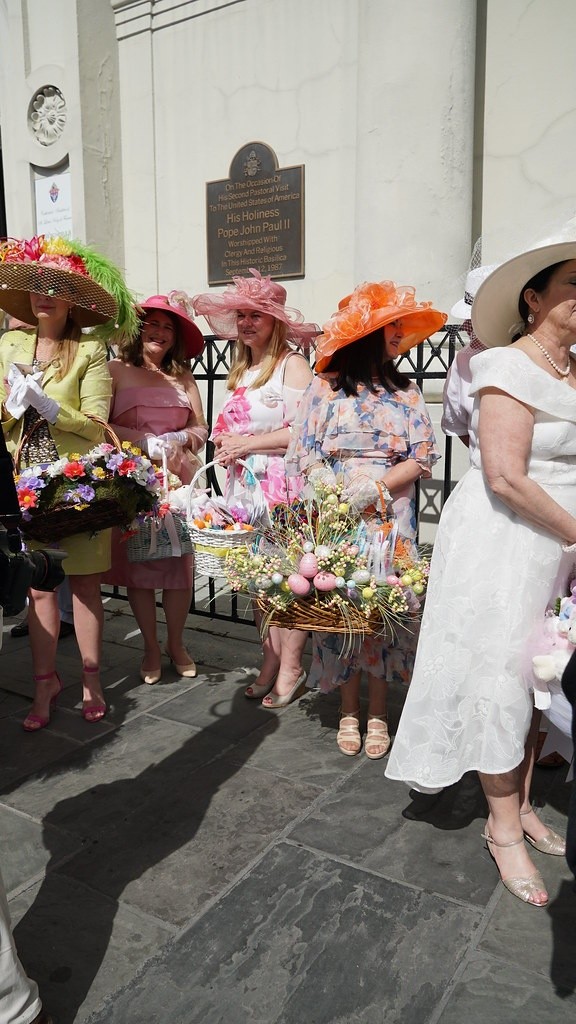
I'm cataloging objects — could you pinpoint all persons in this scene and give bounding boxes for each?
[0,869,54,1024]
[190,268,321,707]
[0,236,141,731]
[285,281,447,759]
[384,221,576,906]
[441,263,501,448]
[100,291,209,683]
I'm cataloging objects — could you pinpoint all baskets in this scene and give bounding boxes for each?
[13,412,142,543]
[125,438,194,562]
[256,590,383,634]
[186,457,265,579]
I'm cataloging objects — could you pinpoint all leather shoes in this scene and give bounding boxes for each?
[141,655,161,685]
[166,645,197,677]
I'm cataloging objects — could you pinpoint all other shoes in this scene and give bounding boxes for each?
[59,621,74,639]
[10,623,30,638]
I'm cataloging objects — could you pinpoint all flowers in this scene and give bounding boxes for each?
[0,234,89,277]
[12,442,171,543]
[168,291,199,319]
[203,468,435,651]
[313,281,426,355]
[234,275,304,329]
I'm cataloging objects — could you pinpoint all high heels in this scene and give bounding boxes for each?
[245,673,278,699]
[262,672,307,708]
[82,666,107,722]
[23,672,63,732]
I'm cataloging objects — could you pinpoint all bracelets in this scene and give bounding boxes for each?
[380,480,389,489]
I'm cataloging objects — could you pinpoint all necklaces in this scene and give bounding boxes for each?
[525,331,570,375]
[142,365,161,372]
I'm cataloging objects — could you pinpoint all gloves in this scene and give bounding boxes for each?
[140,433,170,461]
[157,431,188,446]
[8,366,60,425]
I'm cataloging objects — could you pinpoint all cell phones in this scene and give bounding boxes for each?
[13,362,33,377]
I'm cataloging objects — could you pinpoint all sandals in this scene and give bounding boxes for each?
[364,713,390,759]
[521,805,566,856]
[336,709,361,755]
[481,824,549,907]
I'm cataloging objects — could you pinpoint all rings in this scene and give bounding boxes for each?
[223,451,229,455]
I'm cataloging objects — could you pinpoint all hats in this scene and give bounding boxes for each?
[451,264,501,318]
[315,281,447,378]
[190,270,321,350]
[1,236,146,347]
[132,295,204,360]
[470,241,574,348]
